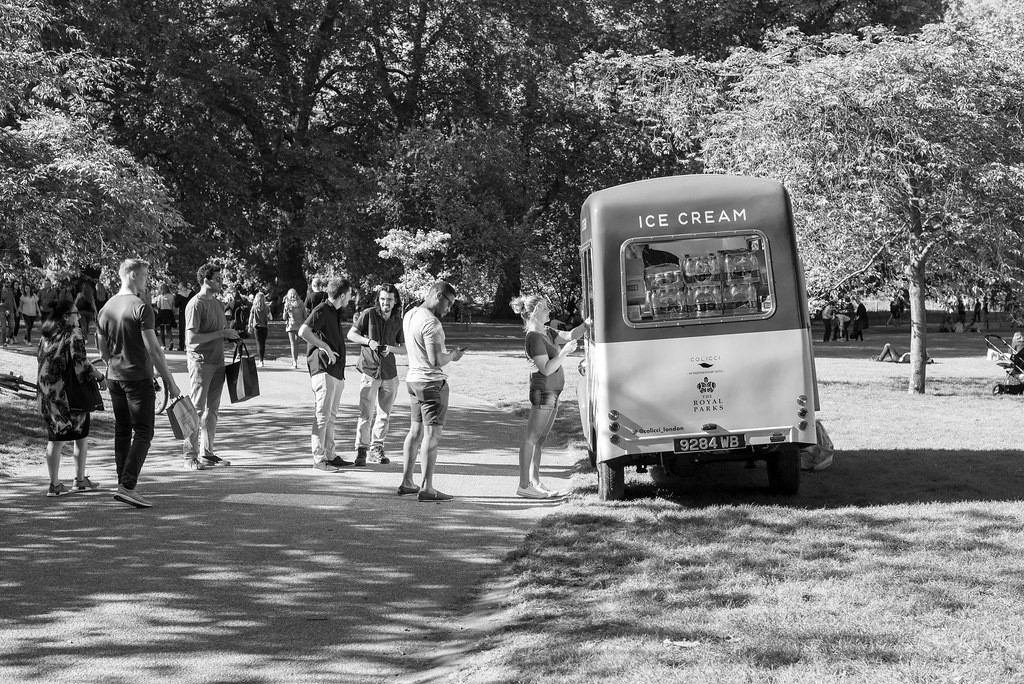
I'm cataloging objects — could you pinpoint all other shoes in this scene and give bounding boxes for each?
[85,339,88,344]
[23,337,33,346]
[13,337,18,344]
[177,347,184,351]
[169,341,173,350]
[161,345,166,350]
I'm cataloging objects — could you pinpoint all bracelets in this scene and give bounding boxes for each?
[97,375,104,382]
[368,339,372,345]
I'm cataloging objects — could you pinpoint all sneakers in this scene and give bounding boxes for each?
[183,458,207,469]
[397,485,420,495]
[326,456,355,467]
[312,460,339,472]
[72,475,100,492]
[47,483,72,496]
[200,456,230,466]
[368,446,390,463]
[354,448,367,465]
[516,481,559,498]
[418,490,454,501]
[113,484,152,506]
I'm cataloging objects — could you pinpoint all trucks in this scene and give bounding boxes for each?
[576,173,820,503]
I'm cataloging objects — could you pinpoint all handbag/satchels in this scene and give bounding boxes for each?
[850,333,856,339]
[225,342,260,403]
[173,322,177,328]
[166,395,202,440]
[64,346,105,414]
[35,311,39,322]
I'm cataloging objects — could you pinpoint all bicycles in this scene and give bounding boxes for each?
[0,371,38,393]
[90,358,168,415]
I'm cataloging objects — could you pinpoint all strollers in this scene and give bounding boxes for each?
[984,334,1024,397]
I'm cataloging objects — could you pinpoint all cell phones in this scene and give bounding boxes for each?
[378,346,387,356]
[459,346,468,352]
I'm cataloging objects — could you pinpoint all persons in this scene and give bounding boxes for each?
[510,294,592,498]
[549,315,567,331]
[346,283,408,466]
[958,301,966,322]
[967,319,979,332]
[398,282,464,503]
[973,299,981,321]
[853,299,869,342]
[952,319,963,333]
[821,298,851,341]
[944,317,954,332]
[0,273,328,370]
[183,263,240,470]
[36,299,107,496]
[298,280,355,473]
[886,298,904,326]
[875,343,933,364]
[97,259,180,507]
[1012,331,1024,350]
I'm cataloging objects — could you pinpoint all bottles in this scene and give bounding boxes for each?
[644,248,760,320]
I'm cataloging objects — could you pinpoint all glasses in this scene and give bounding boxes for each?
[440,292,453,307]
[68,312,80,317]
